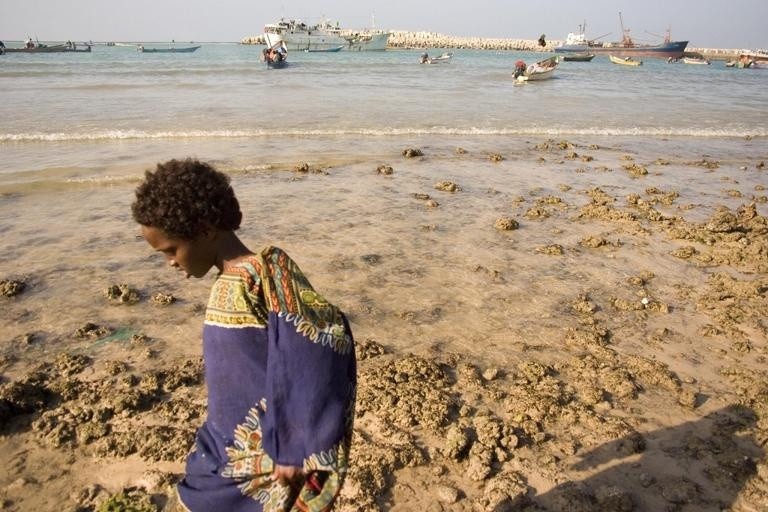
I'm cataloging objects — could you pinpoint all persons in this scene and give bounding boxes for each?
[667,55,681,64]
[262,43,286,63]
[512,58,526,80]
[624,56,633,62]
[131,156,357,512]
[418,51,432,65]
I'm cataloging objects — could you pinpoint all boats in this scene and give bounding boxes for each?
[240,15,392,70]
[422,50,456,65]
[0,39,92,53]
[726,50,767,68]
[681,56,712,66]
[552,10,690,65]
[137,40,201,54]
[512,55,560,87]
